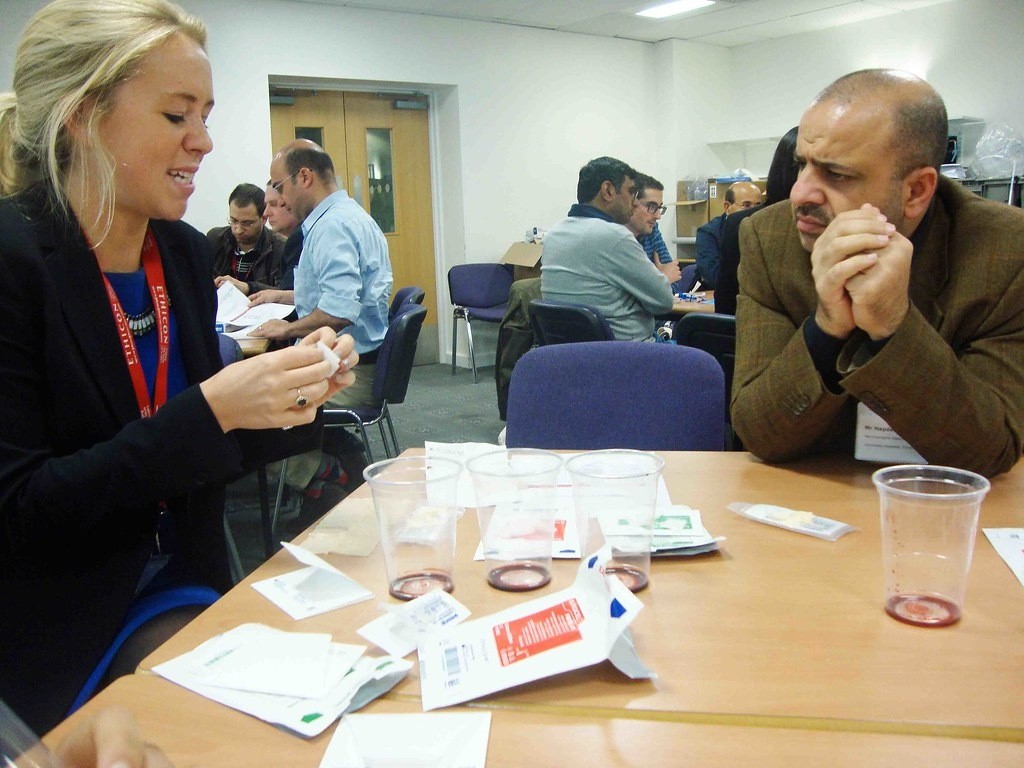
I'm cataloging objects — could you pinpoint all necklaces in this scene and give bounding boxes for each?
[123,292,171,337]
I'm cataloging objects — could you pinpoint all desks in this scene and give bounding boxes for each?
[655,290,715,321]
[0,446,1024,768]
[237,338,271,356]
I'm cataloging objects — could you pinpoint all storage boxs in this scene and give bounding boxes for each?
[500,241,543,281]
[669,180,767,236]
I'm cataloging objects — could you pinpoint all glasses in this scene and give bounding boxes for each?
[272,169,312,199]
[641,200,666,215]
[227,213,261,229]
[735,202,760,210]
[615,187,639,199]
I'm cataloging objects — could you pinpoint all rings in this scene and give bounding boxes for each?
[259,325,262,331]
[295,388,308,407]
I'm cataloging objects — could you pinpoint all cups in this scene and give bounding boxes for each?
[466,449,562,592]
[563,449,665,592]
[362,456,465,600]
[873,465,991,627]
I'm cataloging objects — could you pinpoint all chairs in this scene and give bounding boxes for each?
[216,262,736,586]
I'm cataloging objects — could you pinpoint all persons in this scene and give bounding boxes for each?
[729,69,1024,480]
[0,0,361,713]
[206,183,287,296]
[688,181,761,292]
[715,127,799,315]
[53,709,179,768]
[215,179,304,305]
[248,139,394,534]
[537,156,681,344]
